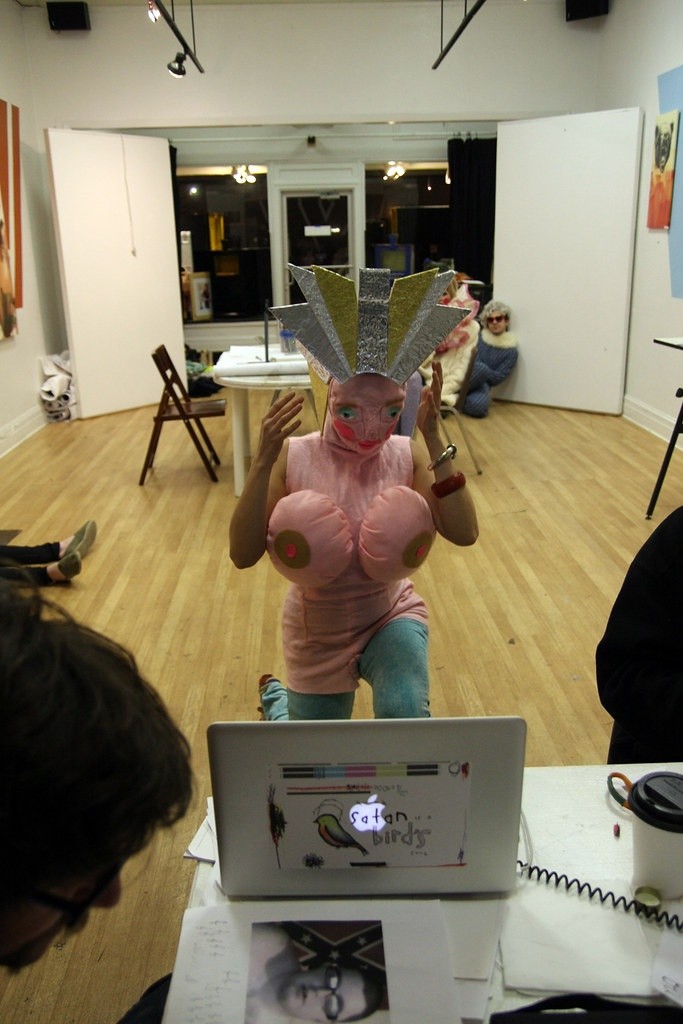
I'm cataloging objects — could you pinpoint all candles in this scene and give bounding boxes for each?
[634,886,662,912]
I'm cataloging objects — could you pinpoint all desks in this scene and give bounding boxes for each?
[211,343,315,498]
[645,337,683,520]
[162,762,683,1024]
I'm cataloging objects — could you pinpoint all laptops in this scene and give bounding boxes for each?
[208,716,527,896]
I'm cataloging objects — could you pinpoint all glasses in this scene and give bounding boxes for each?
[28,870,110,933]
[323,962,344,1024]
[488,315,507,322]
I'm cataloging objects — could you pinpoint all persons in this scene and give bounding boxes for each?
[244,924,382,1024]
[462,300,518,417]
[0,563,196,977]
[595,506,683,764]
[229,361,479,722]
[0,521,97,587]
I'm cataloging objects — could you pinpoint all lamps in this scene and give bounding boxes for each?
[243,165,256,184]
[231,165,239,179]
[148,8,161,23]
[383,162,396,177]
[167,49,187,79]
[396,163,408,175]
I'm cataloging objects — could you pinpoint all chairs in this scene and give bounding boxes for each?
[138,344,226,486]
[399,370,484,475]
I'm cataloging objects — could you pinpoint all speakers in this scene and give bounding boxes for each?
[565,0,609,22]
[46,1,92,31]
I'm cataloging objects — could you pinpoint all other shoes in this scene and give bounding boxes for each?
[64,521,98,560]
[58,551,83,582]
[256,673,283,720]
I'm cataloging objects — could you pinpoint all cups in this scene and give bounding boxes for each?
[628,772,683,899]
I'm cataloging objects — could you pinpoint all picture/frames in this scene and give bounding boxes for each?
[188,271,214,322]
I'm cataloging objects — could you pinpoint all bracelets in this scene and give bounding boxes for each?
[431,472,466,499]
[427,444,457,471]
